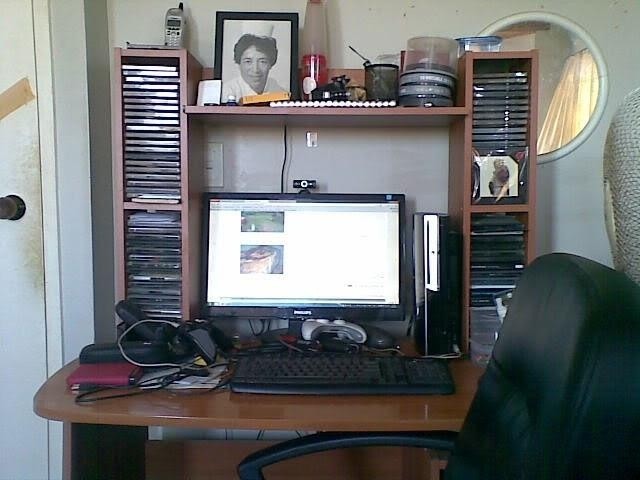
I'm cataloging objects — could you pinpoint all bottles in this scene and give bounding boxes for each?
[299,0,330,100]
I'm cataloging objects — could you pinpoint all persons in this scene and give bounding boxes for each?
[220,33,290,100]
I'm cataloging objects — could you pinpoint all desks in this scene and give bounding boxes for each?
[32,337,486,479]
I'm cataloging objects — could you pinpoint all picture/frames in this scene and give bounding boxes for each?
[212,11,299,102]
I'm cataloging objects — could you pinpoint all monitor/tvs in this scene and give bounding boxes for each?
[197,190,409,356]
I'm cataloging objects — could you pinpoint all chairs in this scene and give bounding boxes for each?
[237,253,640,480]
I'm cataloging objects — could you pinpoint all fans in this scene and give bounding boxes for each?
[603,86,640,285]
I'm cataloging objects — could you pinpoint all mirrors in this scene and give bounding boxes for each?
[474,11,610,165]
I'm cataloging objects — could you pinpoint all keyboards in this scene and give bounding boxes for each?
[231,352,456,396]
